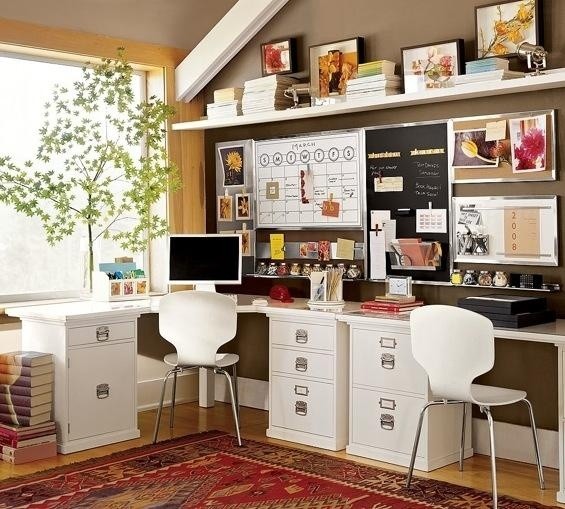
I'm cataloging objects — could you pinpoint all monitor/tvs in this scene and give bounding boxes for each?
[168,233,242,292]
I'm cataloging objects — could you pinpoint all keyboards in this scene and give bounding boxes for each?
[223,294,238,304]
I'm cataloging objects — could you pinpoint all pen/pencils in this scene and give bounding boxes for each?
[107,270,134,280]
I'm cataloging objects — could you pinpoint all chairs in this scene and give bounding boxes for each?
[146,288,246,448]
[402,303,546,505]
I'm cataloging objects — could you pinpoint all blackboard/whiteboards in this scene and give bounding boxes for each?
[254,127,364,232]
[365,119,455,286]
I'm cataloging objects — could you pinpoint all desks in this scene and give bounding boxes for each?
[335,298,565,505]
[4,287,373,456]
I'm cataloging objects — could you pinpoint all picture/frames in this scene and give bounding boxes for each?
[260,36,297,77]
[472,1,544,60]
[308,36,365,96]
[400,38,466,95]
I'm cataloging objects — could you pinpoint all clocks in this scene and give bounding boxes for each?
[383,273,414,301]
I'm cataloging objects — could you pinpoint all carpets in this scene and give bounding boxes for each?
[1,427,562,507]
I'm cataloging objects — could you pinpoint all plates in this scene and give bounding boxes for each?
[306,300,348,308]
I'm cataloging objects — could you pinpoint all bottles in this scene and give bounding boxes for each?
[450,269,463,285]
[492,271,509,286]
[283,83,310,95]
[517,41,549,62]
[478,271,493,285]
[257,262,362,279]
[463,269,479,285]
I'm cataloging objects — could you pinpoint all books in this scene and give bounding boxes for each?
[1,350,57,463]
[455,56,525,86]
[207,74,299,120]
[346,59,402,101]
[361,294,424,312]
